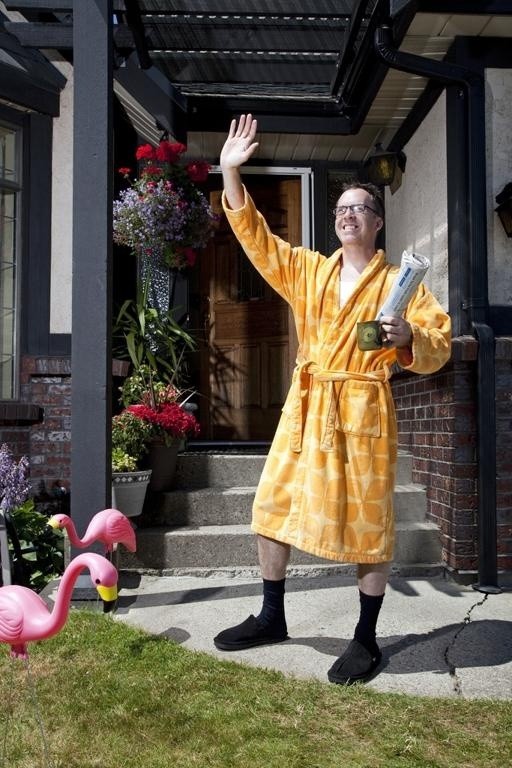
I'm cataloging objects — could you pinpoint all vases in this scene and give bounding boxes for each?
[153,443,183,492]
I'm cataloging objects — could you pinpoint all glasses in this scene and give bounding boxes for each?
[332,204,379,215]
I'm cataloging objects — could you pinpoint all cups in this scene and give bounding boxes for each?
[356,320,391,351]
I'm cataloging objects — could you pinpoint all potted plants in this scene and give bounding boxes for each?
[114,292,199,417]
[109,422,156,519]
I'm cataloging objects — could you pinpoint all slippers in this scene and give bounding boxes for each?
[214,615,287,650]
[328,640,382,685]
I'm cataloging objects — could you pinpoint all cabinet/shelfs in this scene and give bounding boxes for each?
[206,180,302,339]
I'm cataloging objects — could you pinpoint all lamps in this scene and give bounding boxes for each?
[362,137,407,194]
[490,179,512,241]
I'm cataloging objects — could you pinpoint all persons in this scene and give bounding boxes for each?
[214,114,453,684]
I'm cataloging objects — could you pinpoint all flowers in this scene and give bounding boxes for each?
[126,402,201,442]
[0,441,32,513]
[112,138,219,270]
